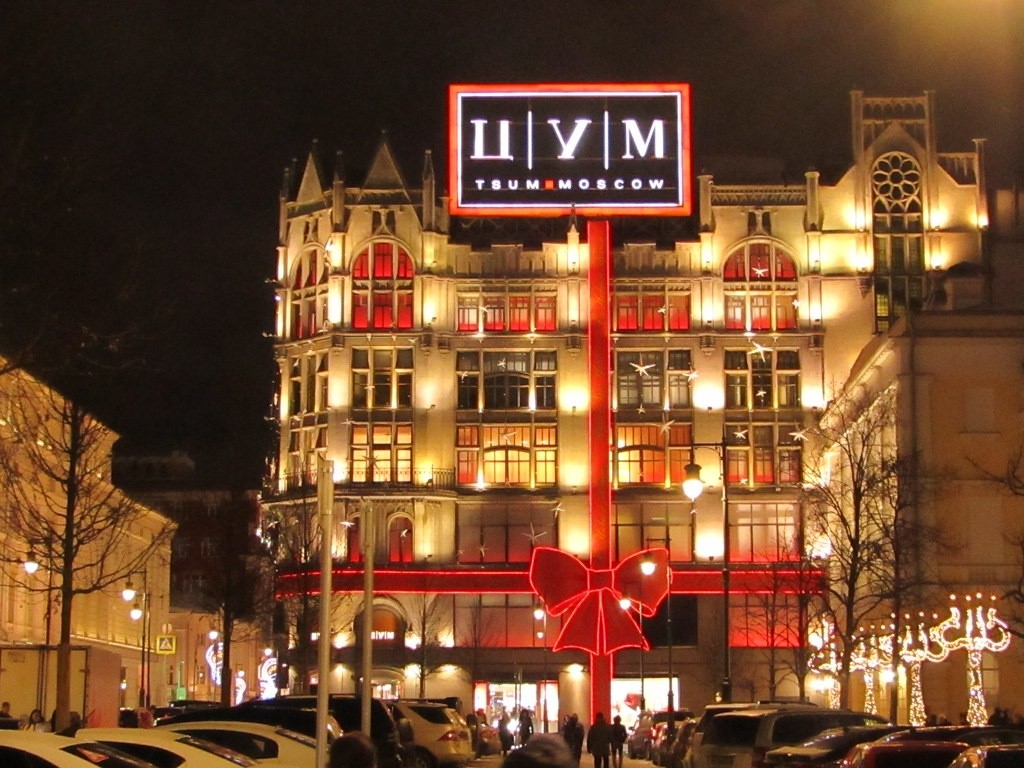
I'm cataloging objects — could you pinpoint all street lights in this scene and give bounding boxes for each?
[676,433,733,702]
[131,590,152,709]
[23,531,54,646]
[122,565,149,708]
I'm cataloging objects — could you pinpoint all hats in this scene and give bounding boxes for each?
[571,712,579,719]
[614,715,621,721]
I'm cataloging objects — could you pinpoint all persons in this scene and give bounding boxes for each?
[497,705,512,754]
[514,709,534,746]
[328,731,379,768]
[609,715,628,768]
[0,701,18,729]
[586,712,610,768]
[562,712,585,768]
[474,707,488,726]
[24,709,51,733]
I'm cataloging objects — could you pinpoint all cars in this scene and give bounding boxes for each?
[0,691,513,768]
[627,699,1024,768]
